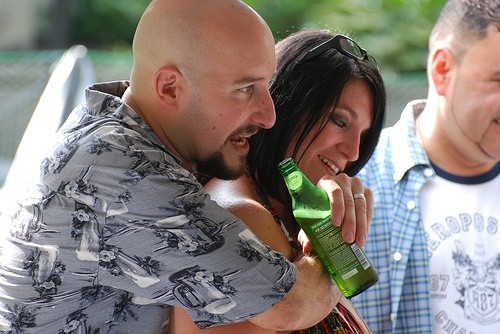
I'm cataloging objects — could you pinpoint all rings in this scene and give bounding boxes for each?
[354,194,366,201]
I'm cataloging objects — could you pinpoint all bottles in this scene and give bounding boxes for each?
[278,158,380,300]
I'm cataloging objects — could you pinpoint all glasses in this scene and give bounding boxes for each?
[298,34,382,79]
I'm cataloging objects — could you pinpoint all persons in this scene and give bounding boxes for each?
[172,27,388,333]
[2,0,373,334]
[332,0,500,334]
